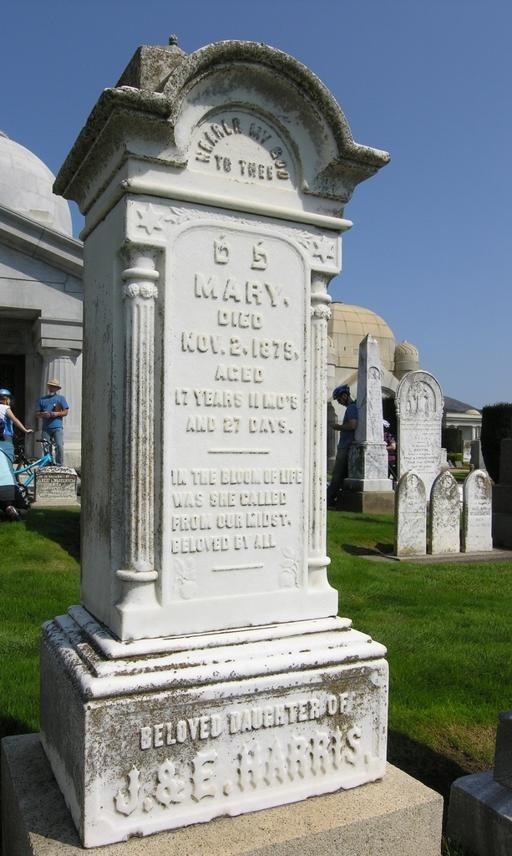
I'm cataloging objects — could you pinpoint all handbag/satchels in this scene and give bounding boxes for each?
[52,403,65,413]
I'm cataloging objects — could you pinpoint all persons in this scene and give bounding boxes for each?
[0,418,34,519]
[383,419,399,488]
[34,376,69,469]
[327,382,359,505]
[0,387,37,463]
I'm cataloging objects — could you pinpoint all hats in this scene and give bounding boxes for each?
[46,378,61,390]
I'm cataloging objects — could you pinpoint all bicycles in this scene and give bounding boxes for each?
[10,437,60,499]
[10,425,41,489]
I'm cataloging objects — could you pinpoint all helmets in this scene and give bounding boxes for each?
[1,387,14,401]
[332,383,351,399]
